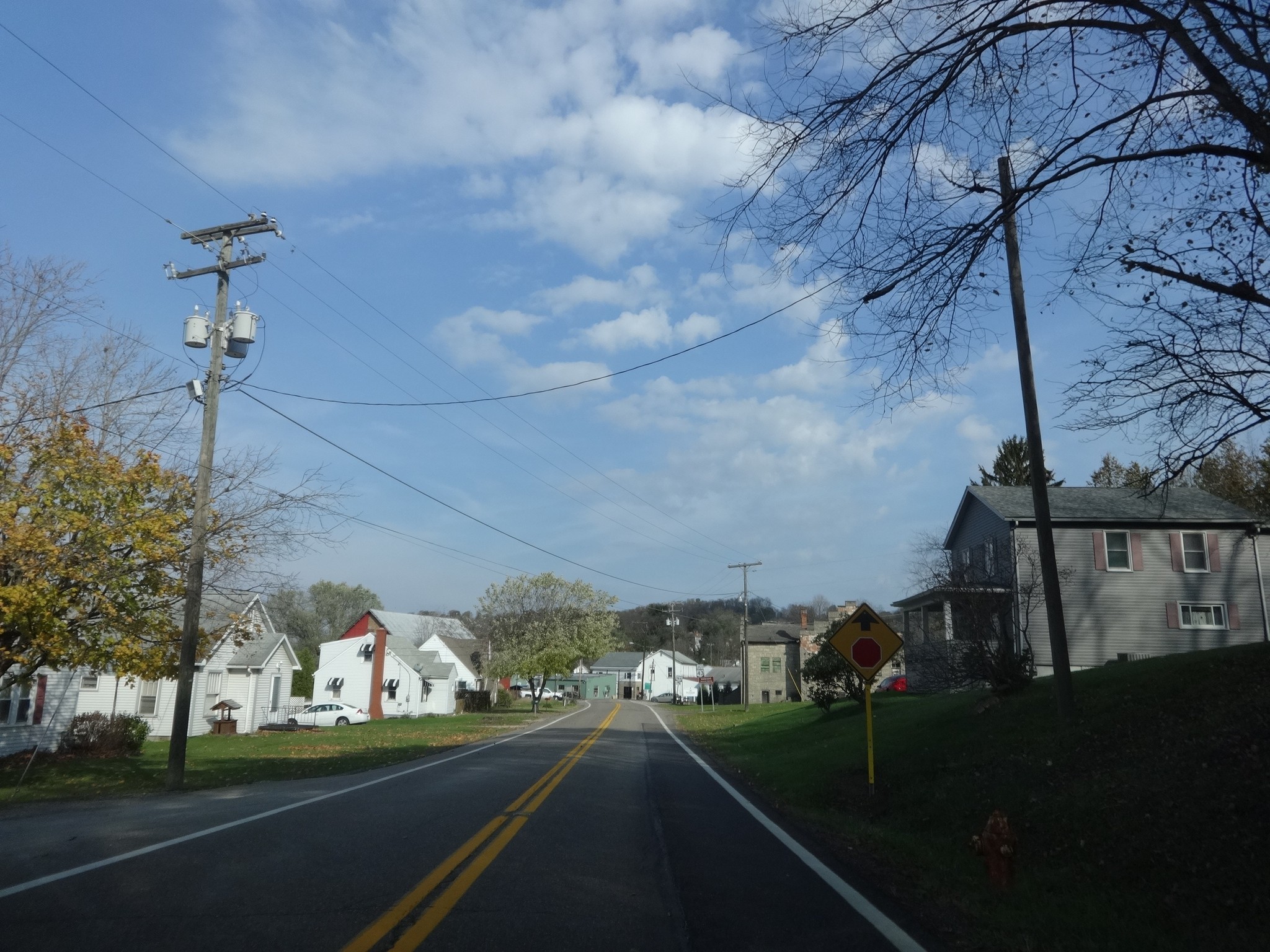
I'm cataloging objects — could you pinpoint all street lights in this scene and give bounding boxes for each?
[665,604,679,705]
[164,300,259,791]
[737,592,752,712]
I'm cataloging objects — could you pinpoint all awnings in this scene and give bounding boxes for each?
[423,679,434,687]
[359,644,375,652]
[384,679,399,688]
[327,677,344,688]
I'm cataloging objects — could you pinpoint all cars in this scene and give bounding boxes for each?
[650,694,681,704]
[287,700,370,726]
[874,674,907,692]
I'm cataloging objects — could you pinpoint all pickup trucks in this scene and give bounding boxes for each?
[520,688,564,702]
